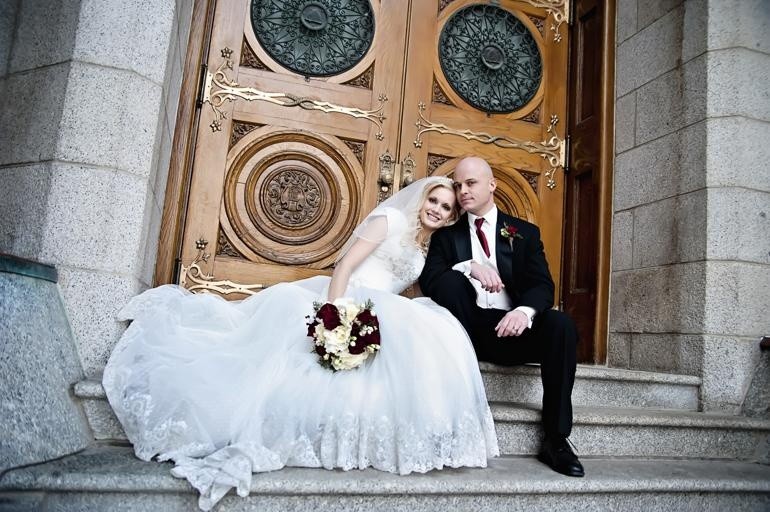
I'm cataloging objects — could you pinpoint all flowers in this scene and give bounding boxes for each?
[497,217,524,253]
[304,295,383,376]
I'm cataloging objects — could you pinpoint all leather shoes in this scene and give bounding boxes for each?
[536,435,587,478]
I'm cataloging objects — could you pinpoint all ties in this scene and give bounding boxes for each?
[473,216,491,260]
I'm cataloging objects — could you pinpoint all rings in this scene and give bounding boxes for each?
[513,326,518,332]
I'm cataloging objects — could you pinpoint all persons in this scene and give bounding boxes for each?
[101,175,500,511]
[417,154,587,478]
